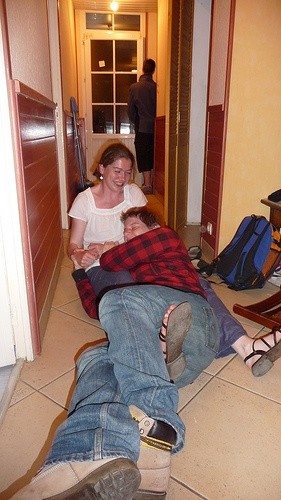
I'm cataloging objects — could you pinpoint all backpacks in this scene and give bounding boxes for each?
[213,214,281,291]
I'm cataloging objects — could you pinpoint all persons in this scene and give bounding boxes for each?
[7,206,220,500]
[66,143,281,384]
[127,59,157,194]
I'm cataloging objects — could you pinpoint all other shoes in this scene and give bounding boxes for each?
[188,246,210,270]
[128,405,177,500]
[7,456,141,500]
[141,184,153,194]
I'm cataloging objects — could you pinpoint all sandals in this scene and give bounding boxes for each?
[243,326,281,377]
[159,300,192,382]
